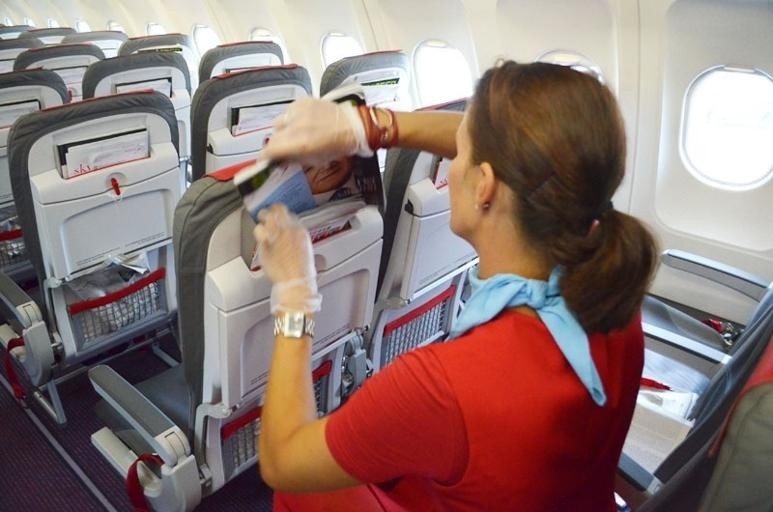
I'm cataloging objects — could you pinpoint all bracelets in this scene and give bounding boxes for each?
[358,104,398,150]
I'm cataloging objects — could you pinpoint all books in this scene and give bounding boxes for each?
[0,99,41,128]
[621,390,698,474]
[54,125,151,180]
[114,77,172,99]
[228,82,386,271]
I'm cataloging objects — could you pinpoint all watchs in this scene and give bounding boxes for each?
[273,312,316,340]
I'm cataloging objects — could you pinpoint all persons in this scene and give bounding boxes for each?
[253,58,663,512]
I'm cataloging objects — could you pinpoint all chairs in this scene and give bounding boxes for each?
[609,309,773,512]
[187,62,311,188]
[89,132,384,512]
[0,21,422,108]
[3,88,183,426]
[367,95,481,376]
[640,237,772,391]
